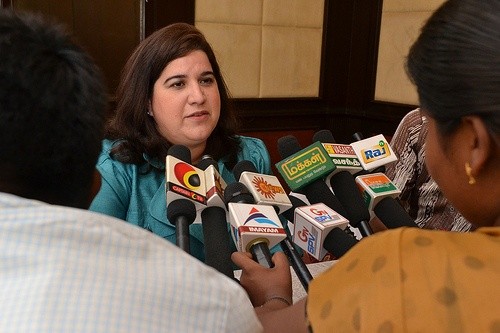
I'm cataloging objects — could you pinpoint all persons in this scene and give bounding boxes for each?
[378,104,474,234]
[0,8,266,333]
[86,23,283,271]
[230,0,500,333]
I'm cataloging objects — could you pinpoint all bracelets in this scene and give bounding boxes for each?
[262,296,291,307]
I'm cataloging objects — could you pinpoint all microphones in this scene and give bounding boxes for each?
[164,129,421,296]
